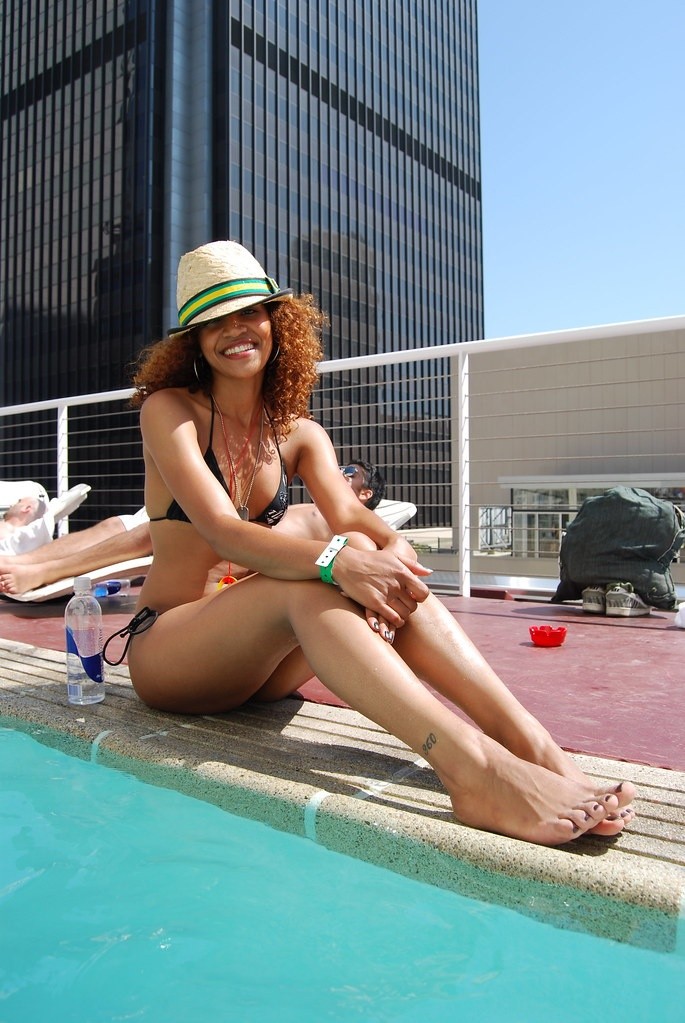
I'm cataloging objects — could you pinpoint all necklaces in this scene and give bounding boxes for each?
[210,393,265,523]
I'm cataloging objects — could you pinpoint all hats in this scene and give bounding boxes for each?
[166,240,295,339]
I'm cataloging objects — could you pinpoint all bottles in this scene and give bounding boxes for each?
[64,577,105,706]
[67,579,131,597]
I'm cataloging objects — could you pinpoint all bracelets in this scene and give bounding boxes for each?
[315,533,348,585]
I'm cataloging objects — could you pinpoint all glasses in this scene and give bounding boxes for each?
[339,465,359,477]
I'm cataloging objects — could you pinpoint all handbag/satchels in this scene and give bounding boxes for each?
[550,486,685,610]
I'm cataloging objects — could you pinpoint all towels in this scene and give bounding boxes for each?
[0,512,55,554]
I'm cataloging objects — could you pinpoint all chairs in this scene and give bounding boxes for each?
[0,498,420,602]
[0,483,91,556]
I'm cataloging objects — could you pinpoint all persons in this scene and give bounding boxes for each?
[0,459,384,595]
[0,497,46,539]
[126,240,637,846]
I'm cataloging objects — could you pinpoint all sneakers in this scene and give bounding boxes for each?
[582,586,605,613]
[605,587,652,616]
